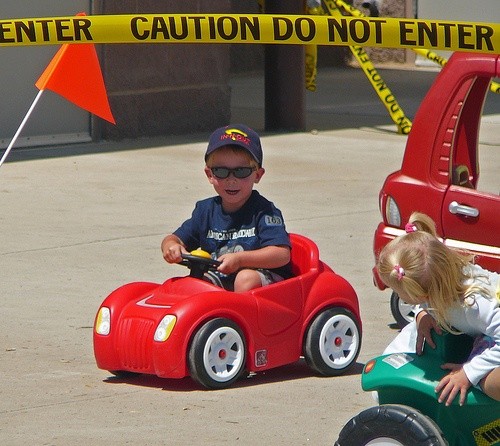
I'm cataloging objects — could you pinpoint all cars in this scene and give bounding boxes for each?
[93,233,364,388]
[334,328,500,446]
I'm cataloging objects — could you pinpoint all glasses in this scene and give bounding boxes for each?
[210,167,257,179]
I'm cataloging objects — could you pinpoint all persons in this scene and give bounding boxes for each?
[160,122,293,292]
[378,212,500,407]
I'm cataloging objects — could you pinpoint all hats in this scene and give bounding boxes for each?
[205,123,262,165]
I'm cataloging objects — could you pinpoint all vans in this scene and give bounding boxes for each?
[370,48,500,326]
